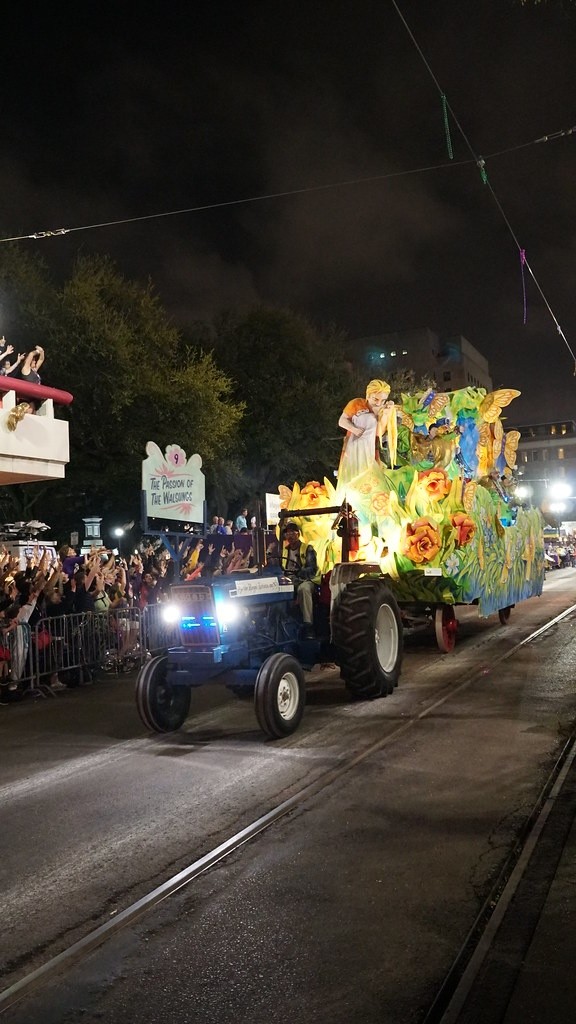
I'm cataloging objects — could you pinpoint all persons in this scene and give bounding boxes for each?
[546,529,576,570]
[489,466,515,504]
[1,509,262,705]
[278,523,323,643]
[338,380,397,482]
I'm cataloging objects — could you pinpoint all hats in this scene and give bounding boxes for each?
[283,523,299,532]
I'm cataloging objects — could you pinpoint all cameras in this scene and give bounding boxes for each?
[112,548,119,556]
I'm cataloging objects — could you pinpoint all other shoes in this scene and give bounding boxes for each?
[0,662,133,706]
[302,625,315,640]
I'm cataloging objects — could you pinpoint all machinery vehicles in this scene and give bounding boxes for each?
[133,495,407,741]
